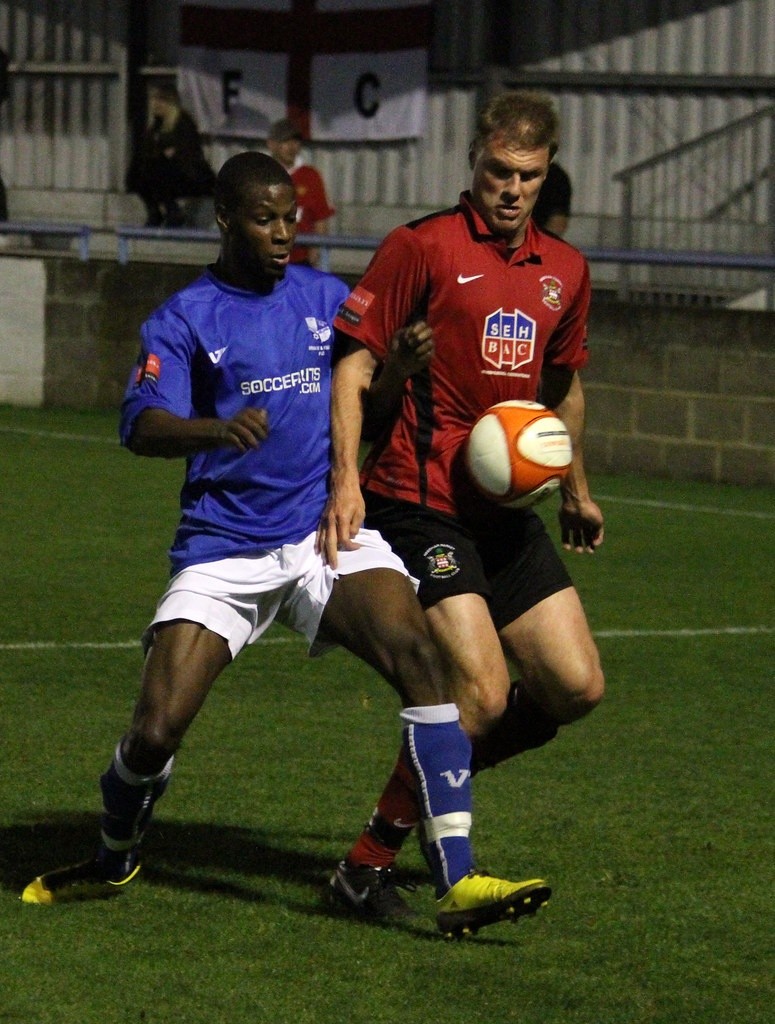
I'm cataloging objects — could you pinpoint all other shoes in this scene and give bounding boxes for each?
[142,211,184,229]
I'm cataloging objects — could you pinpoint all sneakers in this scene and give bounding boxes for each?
[21,853,141,904]
[329,858,434,936]
[435,870,553,942]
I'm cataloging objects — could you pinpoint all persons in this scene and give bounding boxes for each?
[128,75,209,228]
[22,151,552,940]
[263,117,334,273]
[316,90,607,931]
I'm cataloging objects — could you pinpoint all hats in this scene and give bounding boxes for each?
[268,116,303,141]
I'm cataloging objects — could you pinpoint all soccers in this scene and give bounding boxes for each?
[464,398,577,507]
